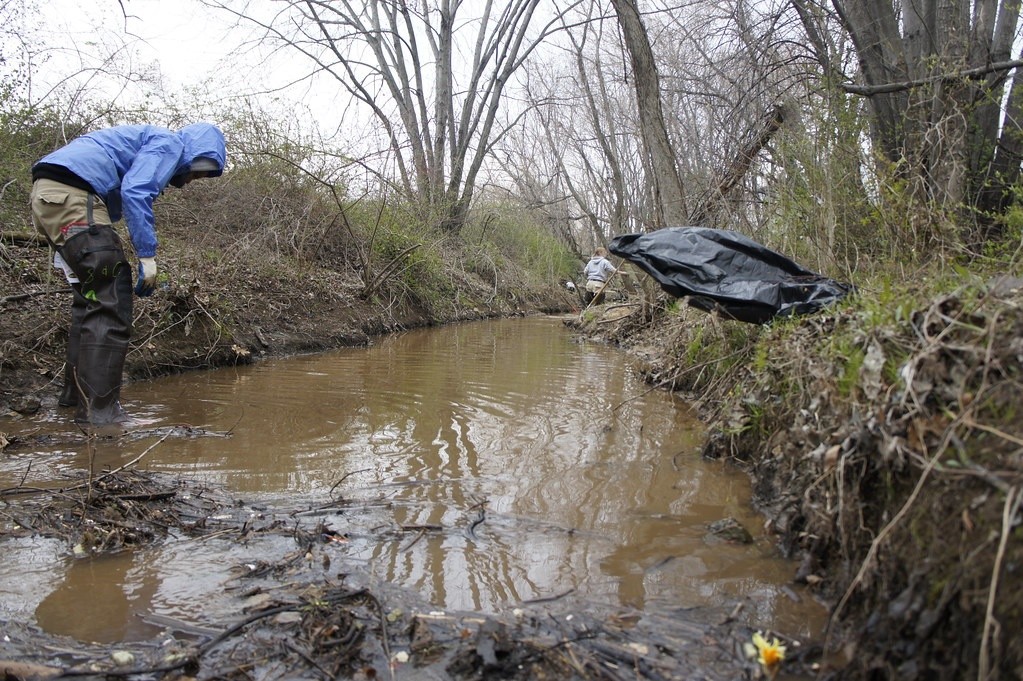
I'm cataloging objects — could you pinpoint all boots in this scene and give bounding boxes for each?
[56,226,134,424]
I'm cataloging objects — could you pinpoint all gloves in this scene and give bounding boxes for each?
[135,257,157,297]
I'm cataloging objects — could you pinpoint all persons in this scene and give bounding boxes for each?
[30,120,227,424]
[583,246,629,306]
